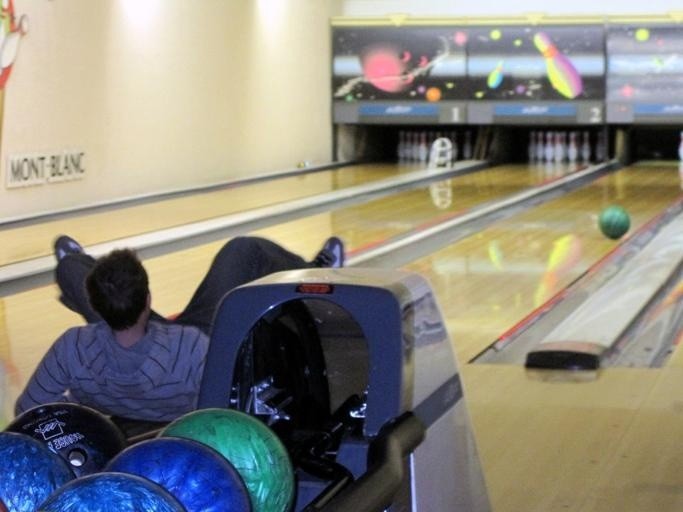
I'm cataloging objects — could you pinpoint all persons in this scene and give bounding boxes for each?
[14,236,344,425]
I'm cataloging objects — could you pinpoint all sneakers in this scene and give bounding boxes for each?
[52,234,82,262]
[312,236,343,267]
